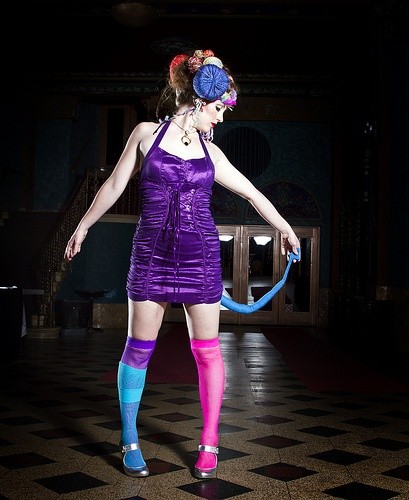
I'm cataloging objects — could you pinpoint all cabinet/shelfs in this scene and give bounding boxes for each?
[87,213,142,329]
[162,225,239,324]
[241,226,319,326]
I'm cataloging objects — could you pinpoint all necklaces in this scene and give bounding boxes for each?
[171,119,198,146]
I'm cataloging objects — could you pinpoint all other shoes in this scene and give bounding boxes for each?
[192,444,219,479]
[119,440,150,477]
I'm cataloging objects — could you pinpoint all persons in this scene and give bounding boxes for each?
[63,49,301,479]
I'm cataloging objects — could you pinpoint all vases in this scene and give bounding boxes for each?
[25,326,61,339]
